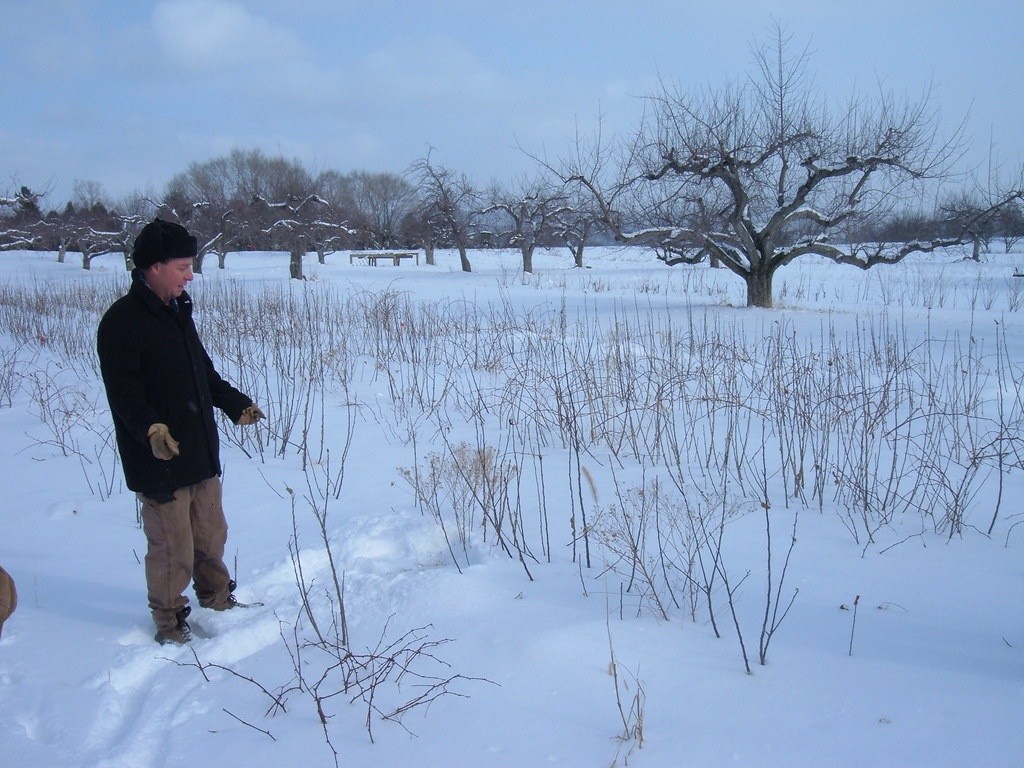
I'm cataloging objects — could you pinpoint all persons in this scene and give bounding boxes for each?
[97,217,269,644]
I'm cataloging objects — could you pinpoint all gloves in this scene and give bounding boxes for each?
[238,403,266,426]
[147,423,179,460]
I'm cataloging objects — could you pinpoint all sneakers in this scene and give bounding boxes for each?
[155,606,192,645]
[211,579,264,611]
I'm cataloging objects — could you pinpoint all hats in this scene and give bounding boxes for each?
[132,217,197,269]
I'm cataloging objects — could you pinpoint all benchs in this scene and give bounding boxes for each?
[357,254,413,267]
